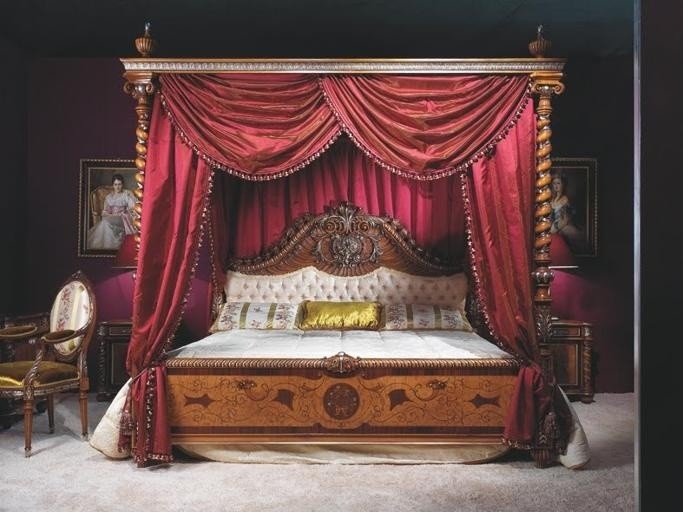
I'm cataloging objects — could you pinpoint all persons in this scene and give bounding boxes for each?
[550,173,578,242]
[87,174,137,250]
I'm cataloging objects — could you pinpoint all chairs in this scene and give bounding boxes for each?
[1,270,97,458]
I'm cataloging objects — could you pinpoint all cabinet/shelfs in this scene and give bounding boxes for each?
[1,310,50,430]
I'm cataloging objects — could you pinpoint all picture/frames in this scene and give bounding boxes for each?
[75,157,138,264]
[549,156,600,260]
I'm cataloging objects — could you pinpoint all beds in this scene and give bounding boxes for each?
[87,200,593,471]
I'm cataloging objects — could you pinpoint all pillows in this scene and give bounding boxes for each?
[207,298,474,333]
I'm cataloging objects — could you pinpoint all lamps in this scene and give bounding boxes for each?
[547,233,580,322]
[112,233,139,320]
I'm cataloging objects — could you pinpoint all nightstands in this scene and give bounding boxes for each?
[550,320,594,404]
[96,318,134,403]
